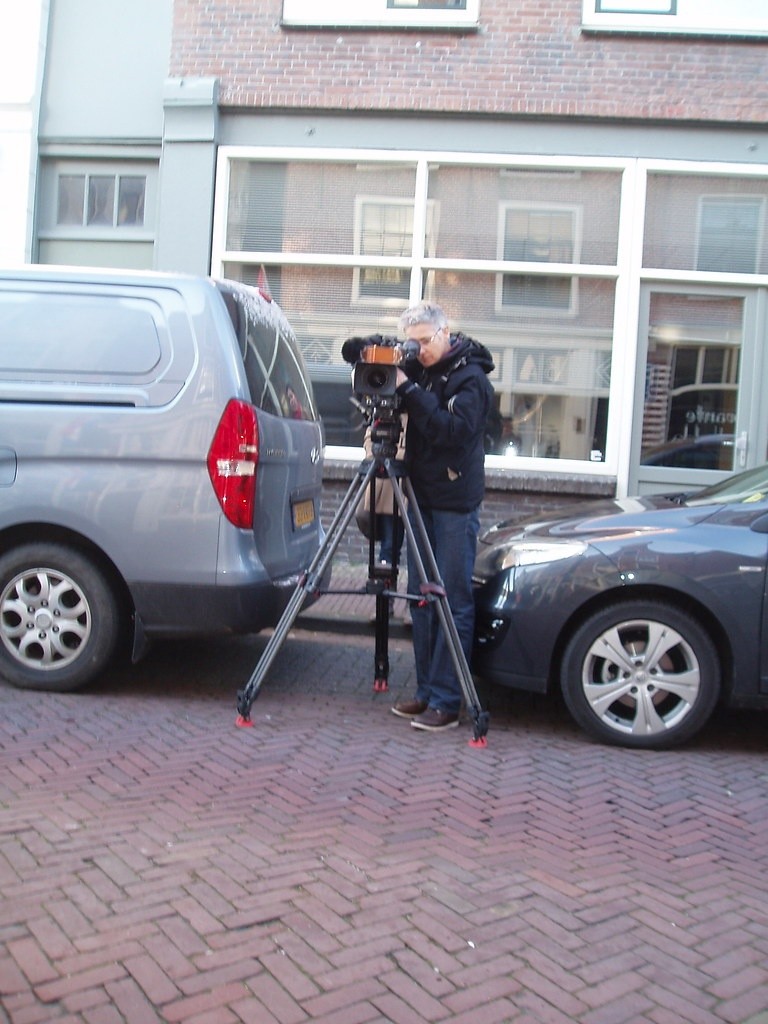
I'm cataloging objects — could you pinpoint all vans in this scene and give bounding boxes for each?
[0,267,330,693]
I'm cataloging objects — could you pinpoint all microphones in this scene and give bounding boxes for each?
[340,335,375,364]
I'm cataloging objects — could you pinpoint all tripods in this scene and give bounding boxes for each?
[234,410,487,749]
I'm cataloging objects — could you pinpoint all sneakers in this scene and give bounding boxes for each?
[391,699,427,718]
[411,708,458,731]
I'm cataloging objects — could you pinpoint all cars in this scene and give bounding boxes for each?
[467,463,767,753]
[643,433,737,469]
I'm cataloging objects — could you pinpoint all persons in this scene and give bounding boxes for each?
[284,383,311,421]
[666,406,701,443]
[364,301,502,732]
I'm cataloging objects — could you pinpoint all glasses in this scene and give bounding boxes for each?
[419,328,442,347]
